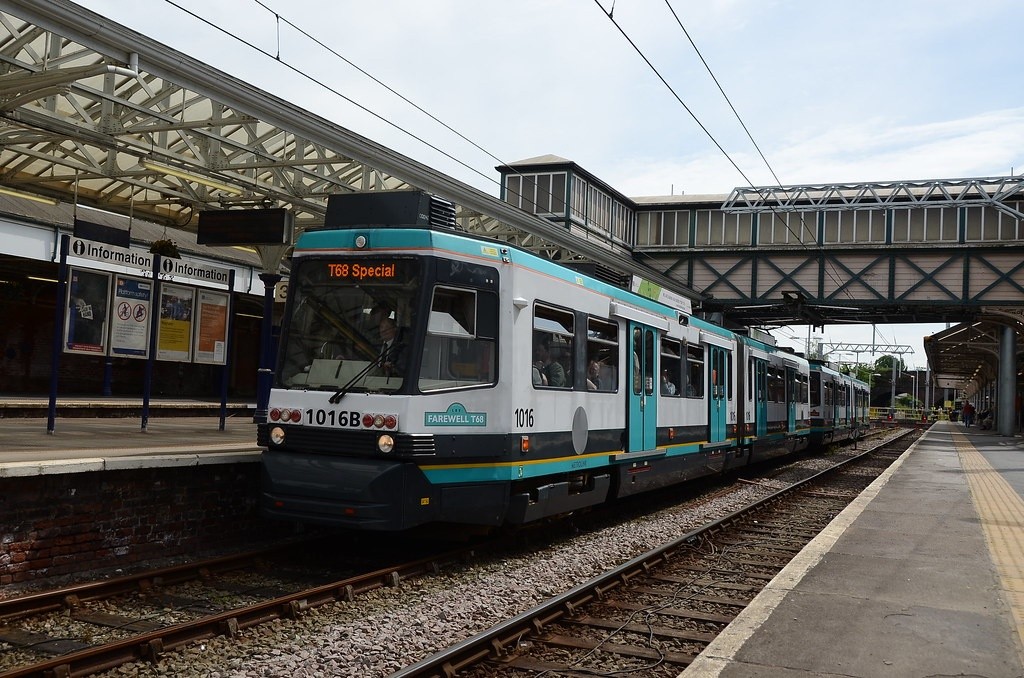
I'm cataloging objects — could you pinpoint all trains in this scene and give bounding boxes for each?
[236,189,875,542]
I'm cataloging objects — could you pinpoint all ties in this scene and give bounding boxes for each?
[381,343,388,375]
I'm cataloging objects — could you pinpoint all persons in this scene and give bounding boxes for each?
[161,296,191,321]
[661,370,675,396]
[334,317,409,378]
[533,341,566,387]
[931,406,959,422]
[567,360,602,390]
[960,400,994,430]
